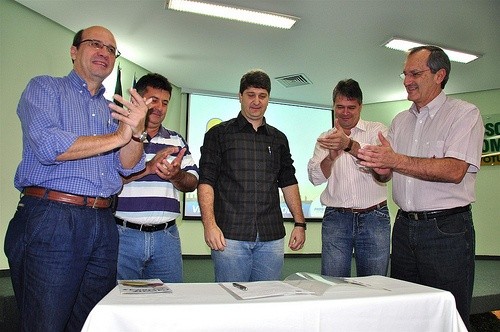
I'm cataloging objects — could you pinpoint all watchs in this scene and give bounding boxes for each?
[132,130,147,141]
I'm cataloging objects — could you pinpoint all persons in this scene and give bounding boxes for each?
[198,70,306,282]
[356,46,485,332]
[307,79,391,278]
[112,72,200,283]
[4,25,153,329]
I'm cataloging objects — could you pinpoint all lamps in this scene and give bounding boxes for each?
[379,36,481,64]
[165,0,301,31]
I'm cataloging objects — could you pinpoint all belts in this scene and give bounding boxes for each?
[400,203,472,220]
[112,214,175,232]
[334,200,387,215]
[22,186,112,209]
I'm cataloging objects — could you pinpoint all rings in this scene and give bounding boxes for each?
[168,171,170,176]
[127,109,131,117]
[331,143,333,147]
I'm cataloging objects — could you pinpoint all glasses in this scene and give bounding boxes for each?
[400,69,431,81]
[74,39,121,58]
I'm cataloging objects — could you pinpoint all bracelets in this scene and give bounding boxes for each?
[294,223,307,230]
[343,138,353,152]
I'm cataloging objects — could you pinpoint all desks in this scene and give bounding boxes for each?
[80,275,468,332]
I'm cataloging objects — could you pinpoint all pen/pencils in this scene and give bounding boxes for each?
[232,282,248,291]
